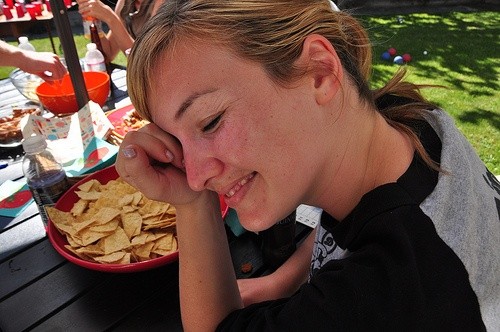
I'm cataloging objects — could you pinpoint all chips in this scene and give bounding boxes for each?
[45,176,177,267]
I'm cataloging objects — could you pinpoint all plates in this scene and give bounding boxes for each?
[55,134,120,179]
[106,105,138,135]
[0,107,54,148]
[46,165,232,273]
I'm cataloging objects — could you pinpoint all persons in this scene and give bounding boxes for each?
[115,0,500,332]
[0,41,67,83]
[77,1,172,63]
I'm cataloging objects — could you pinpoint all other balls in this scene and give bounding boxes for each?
[423,51,428,55]
[380,46,411,65]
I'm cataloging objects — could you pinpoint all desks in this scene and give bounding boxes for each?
[0,61,320,332]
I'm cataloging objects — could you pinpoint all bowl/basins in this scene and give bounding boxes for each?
[10,58,84,105]
[37,71,110,115]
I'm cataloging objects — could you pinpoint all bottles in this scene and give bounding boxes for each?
[84,43,107,72]
[18,36,36,52]
[22,135,68,230]
[89,24,111,74]
